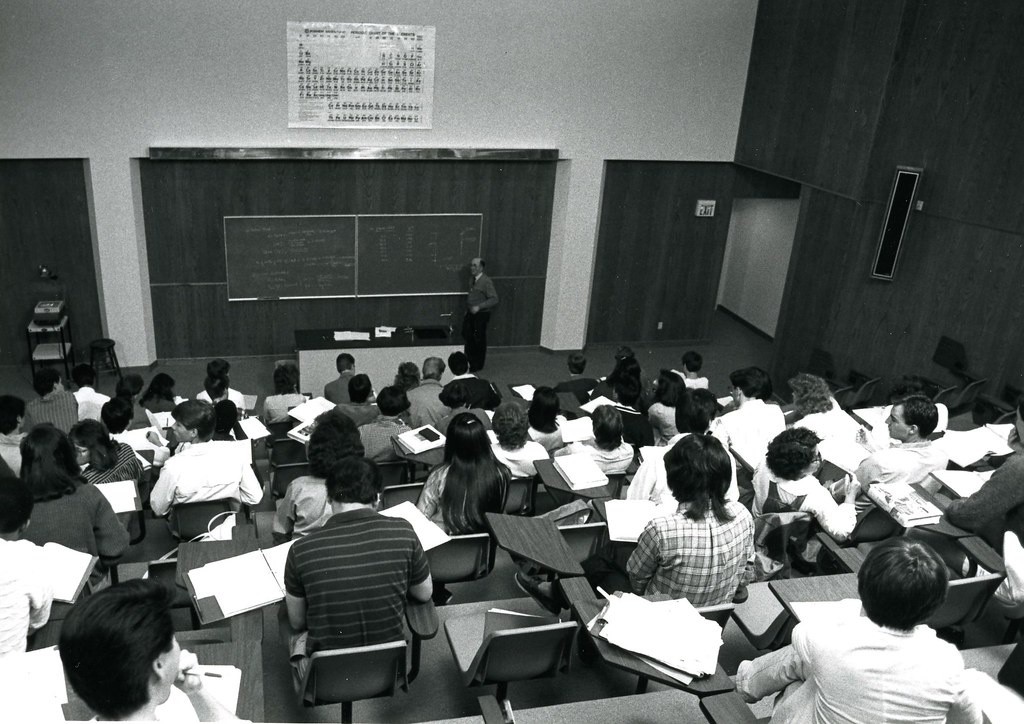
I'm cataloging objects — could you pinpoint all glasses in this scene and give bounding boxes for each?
[647,378,659,393]
[811,452,823,464]
[729,388,741,397]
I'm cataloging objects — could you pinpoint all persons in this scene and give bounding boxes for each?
[0,346,1024,723]
[462,258,499,373]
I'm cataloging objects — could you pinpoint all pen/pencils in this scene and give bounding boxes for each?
[203,672,222,678]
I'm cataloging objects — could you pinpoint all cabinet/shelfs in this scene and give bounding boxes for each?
[27,313,75,389]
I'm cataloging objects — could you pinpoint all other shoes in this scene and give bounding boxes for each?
[789,550,818,576]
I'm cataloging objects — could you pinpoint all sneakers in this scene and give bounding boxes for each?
[515,570,561,617]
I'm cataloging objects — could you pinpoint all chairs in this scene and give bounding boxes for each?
[270,378,1024,649]
[167,497,234,540]
[444,608,579,705]
[288,636,410,724]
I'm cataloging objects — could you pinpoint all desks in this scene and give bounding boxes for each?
[860,483,977,538]
[293,325,466,398]
[390,437,445,466]
[27,395,265,723]
[490,382,503,398]
[554,392,586,420]
[507,384,538,398]
[574,593,736,696]
[485,512,585,575]
[769,574,863,622]
[534,458,610,499]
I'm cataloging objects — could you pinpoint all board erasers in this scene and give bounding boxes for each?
[257,293,279,300]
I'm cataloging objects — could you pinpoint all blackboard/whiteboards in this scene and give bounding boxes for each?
[223,213,484,302]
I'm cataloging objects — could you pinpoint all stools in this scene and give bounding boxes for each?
[88,339,123,392]
